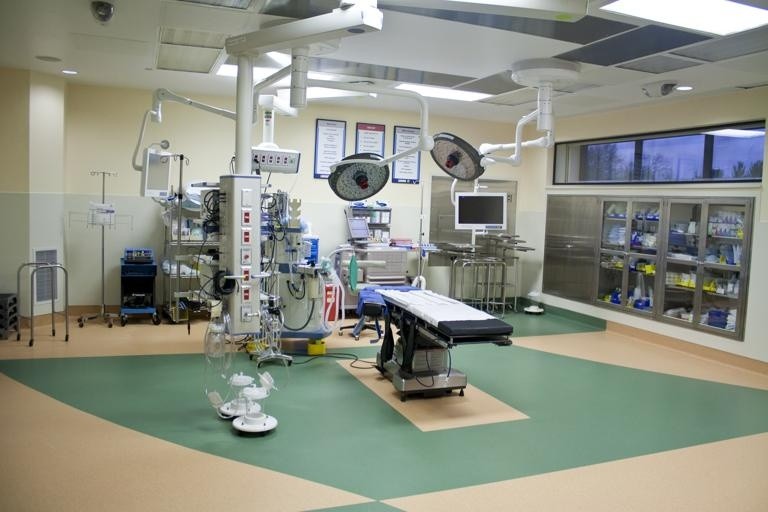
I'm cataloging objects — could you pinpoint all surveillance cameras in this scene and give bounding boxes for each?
[90,1,115,23]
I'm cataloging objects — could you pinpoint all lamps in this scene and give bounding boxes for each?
[225,0,382,56]
[430,78,554,181]
[586,0,768,38]
[328,136,433,201]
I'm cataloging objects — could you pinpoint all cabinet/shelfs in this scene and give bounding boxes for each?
[594,198,758,340]
[119,258,159,324]
[162,197,219,323]
[343,206,392,242]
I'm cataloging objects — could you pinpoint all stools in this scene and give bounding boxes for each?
[359,291,386,334]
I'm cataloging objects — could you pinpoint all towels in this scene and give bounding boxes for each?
[349,255,358,291]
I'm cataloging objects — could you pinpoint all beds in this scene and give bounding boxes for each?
[374,283,517,402]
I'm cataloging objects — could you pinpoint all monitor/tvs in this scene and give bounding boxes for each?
[140,147,172,198]
[454,191,507,231]
[347,217,371,239]
